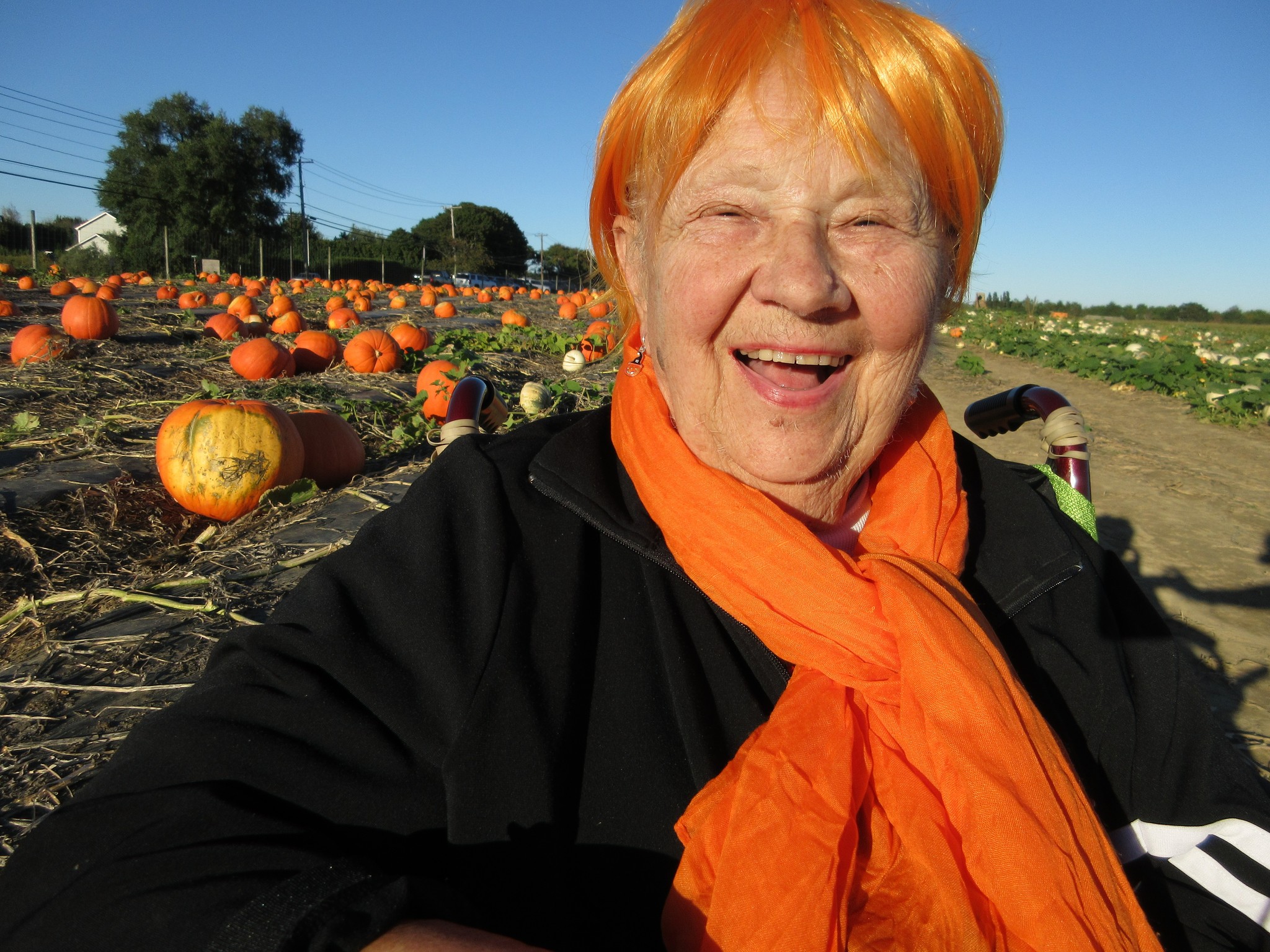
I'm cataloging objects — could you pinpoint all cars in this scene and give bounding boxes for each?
[292,273,321,281]
[488,276,520,290]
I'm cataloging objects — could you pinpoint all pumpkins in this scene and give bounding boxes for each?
[0,263,623,381]
[417,360,462,425]
[934,308,1269,409]
[287,411,366,484]
[519,382,551,415]
[155,396,306,524]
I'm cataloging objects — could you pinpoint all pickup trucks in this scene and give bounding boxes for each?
[411,269,454,287]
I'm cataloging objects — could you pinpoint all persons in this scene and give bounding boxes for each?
[0,3,1270,949]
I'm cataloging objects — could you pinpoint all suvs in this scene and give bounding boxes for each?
[516,278,551,292]
[453,273,497,287]
